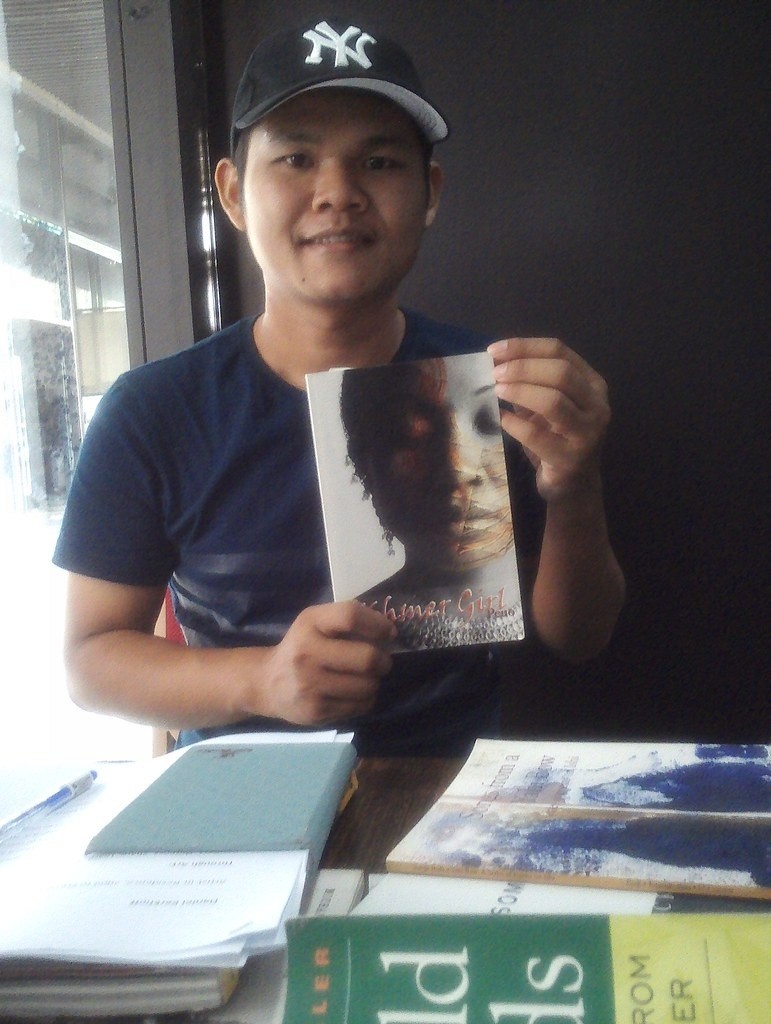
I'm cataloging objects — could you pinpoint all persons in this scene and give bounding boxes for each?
[341,352,525,653]
[50,17,627,757]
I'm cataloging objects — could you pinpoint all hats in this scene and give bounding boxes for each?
[230,21,449,157]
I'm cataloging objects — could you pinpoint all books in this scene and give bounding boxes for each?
[305,352,524,653]
[281,913,771,1024]
[0,968,239,1019]
[386,738,771,904]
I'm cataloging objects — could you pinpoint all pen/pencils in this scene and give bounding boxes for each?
[0,769,96,847]
